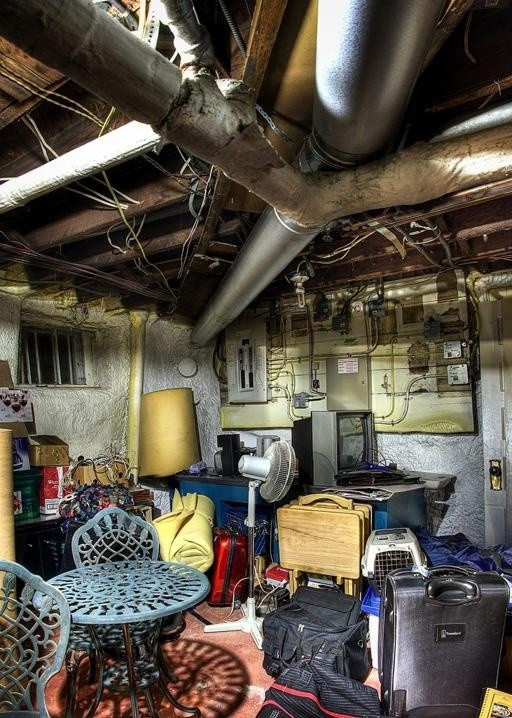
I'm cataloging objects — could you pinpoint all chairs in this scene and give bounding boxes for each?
[72,505,181,685]
[1,560,72,717]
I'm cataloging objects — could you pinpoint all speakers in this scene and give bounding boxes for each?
[256,435,280,457]
[214,434,240,476]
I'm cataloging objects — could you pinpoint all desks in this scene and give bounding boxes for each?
[175,473,429,565]
[33,560,212,716]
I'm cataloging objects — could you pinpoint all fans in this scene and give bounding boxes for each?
[203,440,298,652]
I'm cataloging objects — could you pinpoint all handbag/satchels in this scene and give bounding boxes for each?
[255,661,381,718]
[263,603,369,680]
[293,587,362,629]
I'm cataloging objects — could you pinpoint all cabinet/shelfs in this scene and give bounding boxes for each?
[17,495,164,617]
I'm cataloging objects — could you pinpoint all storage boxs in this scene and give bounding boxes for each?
[13,432,131,521]
[305,577,335,592]
[360,585,383,674]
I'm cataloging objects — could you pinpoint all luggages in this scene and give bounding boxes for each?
[207,527,247,605]
[378,567,510,718]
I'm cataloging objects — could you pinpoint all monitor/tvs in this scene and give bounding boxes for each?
[291,411,372,487]
